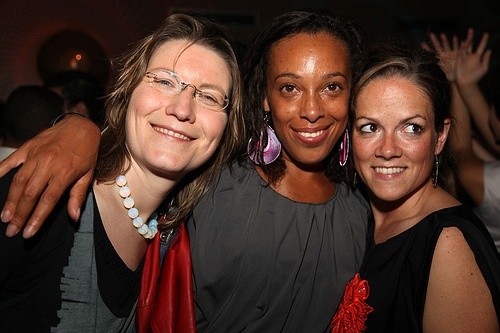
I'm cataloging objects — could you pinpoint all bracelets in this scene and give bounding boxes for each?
[48,111,85,126]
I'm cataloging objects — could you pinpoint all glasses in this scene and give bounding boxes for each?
[144,69,229,111]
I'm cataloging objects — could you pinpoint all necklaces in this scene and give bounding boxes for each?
[115,174,159,239]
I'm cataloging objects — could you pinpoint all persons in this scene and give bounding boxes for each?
[0,13,248,333]
[0,70,112,148]
[347,48,500,333]
[0,11,370,333]
[420,28,500,254]
